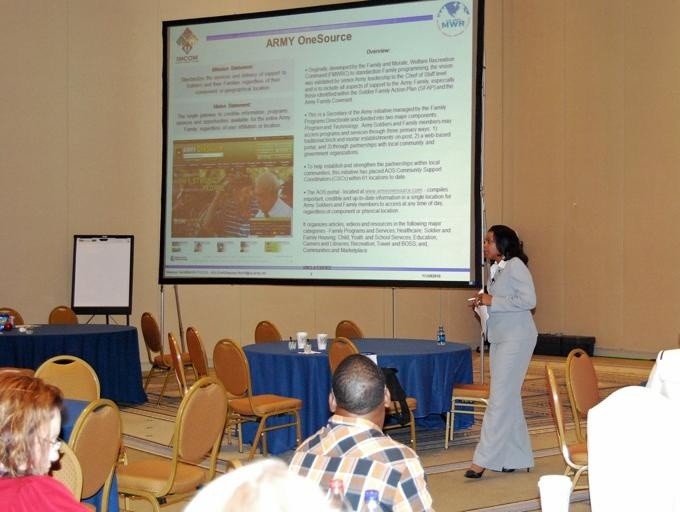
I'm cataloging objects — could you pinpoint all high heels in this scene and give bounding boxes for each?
[492,466,530,473]
[464,467,485,478]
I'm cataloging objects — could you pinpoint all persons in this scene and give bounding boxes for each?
[288,353,432,512]
[0,369,89,511]
[464,225,538,479]
[201,174,291,236]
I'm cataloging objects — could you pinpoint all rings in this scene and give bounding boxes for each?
[477,301,481,304]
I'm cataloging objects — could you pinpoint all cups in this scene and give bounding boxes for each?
[537,473,575,512]
[316,333,329,351]
[296,331,308,351]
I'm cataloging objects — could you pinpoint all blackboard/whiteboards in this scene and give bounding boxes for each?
[71,237,133,310]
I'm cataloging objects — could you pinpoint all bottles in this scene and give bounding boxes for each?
[436,326,446,346]
[288,337,297,351]
[303,338,312,353]
[0,312,16,328]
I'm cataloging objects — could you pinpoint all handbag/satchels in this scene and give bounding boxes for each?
[383,367,406,401]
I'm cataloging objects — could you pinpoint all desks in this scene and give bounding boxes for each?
[0,325,149,407]
[232,339,474,439]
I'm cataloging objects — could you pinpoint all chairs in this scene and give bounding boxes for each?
[31,353,101,406]
[214,340,305,460]
[440,378,491,450]
[333,318,367,338]
[45,306,81,325]
[327,341,418,457]
[64,397,123,511]
[544,364,590,494]
[249,319,285,343]
[0,306,25,326]
[117,375,229,512]
[137,313,216,443]
[564,348,597,442]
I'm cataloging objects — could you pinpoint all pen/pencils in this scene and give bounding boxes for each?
[466,297,475,302]
